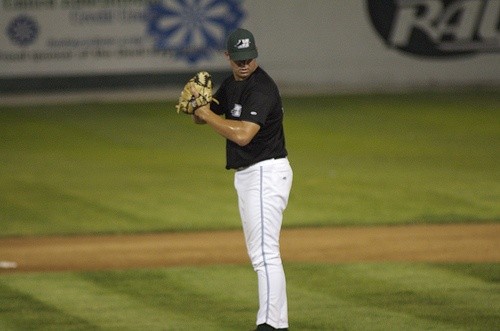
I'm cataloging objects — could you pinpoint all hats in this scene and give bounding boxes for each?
[227,28,258,61]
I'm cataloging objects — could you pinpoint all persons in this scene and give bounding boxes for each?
[182,28,294,331]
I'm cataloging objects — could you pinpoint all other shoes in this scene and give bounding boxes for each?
[257,324,288,331]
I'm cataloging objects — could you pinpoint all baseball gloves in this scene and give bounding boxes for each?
[178,71,214,113]
